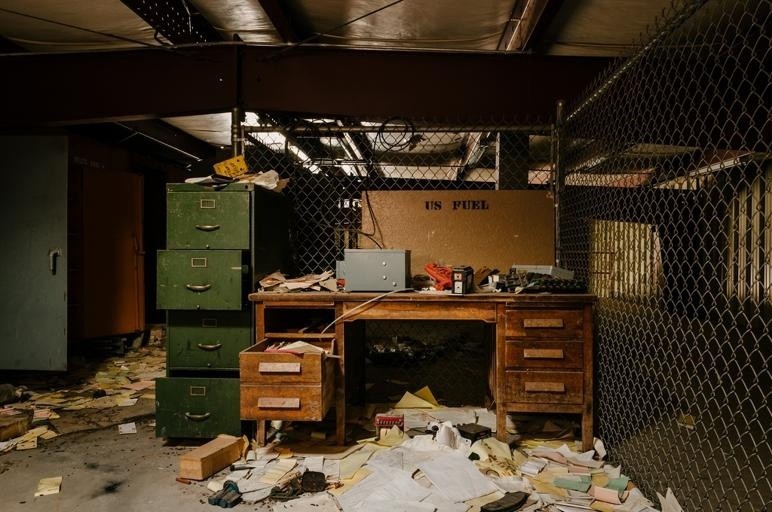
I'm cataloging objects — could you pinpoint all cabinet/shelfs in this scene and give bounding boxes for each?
[0,133,146,372]
[156,183,257,438]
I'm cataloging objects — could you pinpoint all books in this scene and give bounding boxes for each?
[0,345,167,453]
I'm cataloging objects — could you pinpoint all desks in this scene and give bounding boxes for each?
[238,287,598,450]
